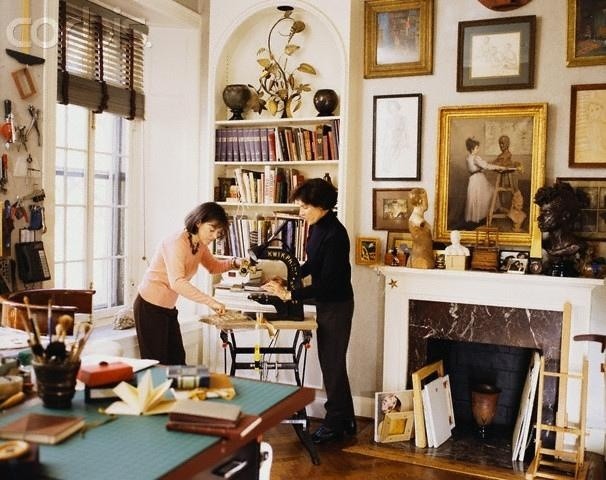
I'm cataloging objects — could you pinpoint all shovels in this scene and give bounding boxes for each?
[0,200,16,293]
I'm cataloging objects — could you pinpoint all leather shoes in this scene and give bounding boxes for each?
[343,421,358,435]
[312,422,346,444]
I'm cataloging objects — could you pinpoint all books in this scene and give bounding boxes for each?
[213,118,340,262]
[0,364,262,447]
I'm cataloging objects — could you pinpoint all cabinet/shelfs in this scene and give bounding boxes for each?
[216,115,340,288]
[211,297,323,425]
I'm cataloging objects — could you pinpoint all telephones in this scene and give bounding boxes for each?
[15,241,51,284]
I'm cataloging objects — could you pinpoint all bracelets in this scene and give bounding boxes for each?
[283,290,289,303]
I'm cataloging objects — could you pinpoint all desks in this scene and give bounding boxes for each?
[375,265,605,458]
[0,354,315,480]
[198,312,320,466]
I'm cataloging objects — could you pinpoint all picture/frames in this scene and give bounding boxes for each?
[433,103,547,245]
[386,232,413,254]
[363,0,434,79]
[566,0,606,67]
[372,188,414,231]
[456,14,536,92]
[556,176,606,242]
[507,258,528,274]
[499,250,529,272]
[372,92,422,181]
[568,83,606,168]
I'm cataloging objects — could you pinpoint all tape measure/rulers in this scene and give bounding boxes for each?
[5,0,45,66]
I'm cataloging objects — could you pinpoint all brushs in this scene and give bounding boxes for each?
[23,189,45,202]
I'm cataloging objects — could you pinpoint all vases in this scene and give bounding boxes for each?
[313,89,337,116]
[222,84,250,120]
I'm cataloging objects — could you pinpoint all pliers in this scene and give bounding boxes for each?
[24,105,41,147]
[17,126,27,152]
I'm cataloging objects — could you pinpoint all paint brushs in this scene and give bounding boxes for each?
[18,295,90,364]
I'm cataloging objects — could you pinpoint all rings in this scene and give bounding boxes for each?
[272,290,275,293]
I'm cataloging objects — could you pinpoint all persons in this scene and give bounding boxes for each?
[444,229,471,257]
[132,202,250,366]
[406,187,435,269]
[500,252,528,272]
[490,135,512,167]
[463,137,506,229]
[507,191,527,225]
[259,177,358,445]
[532,182,587,258]
